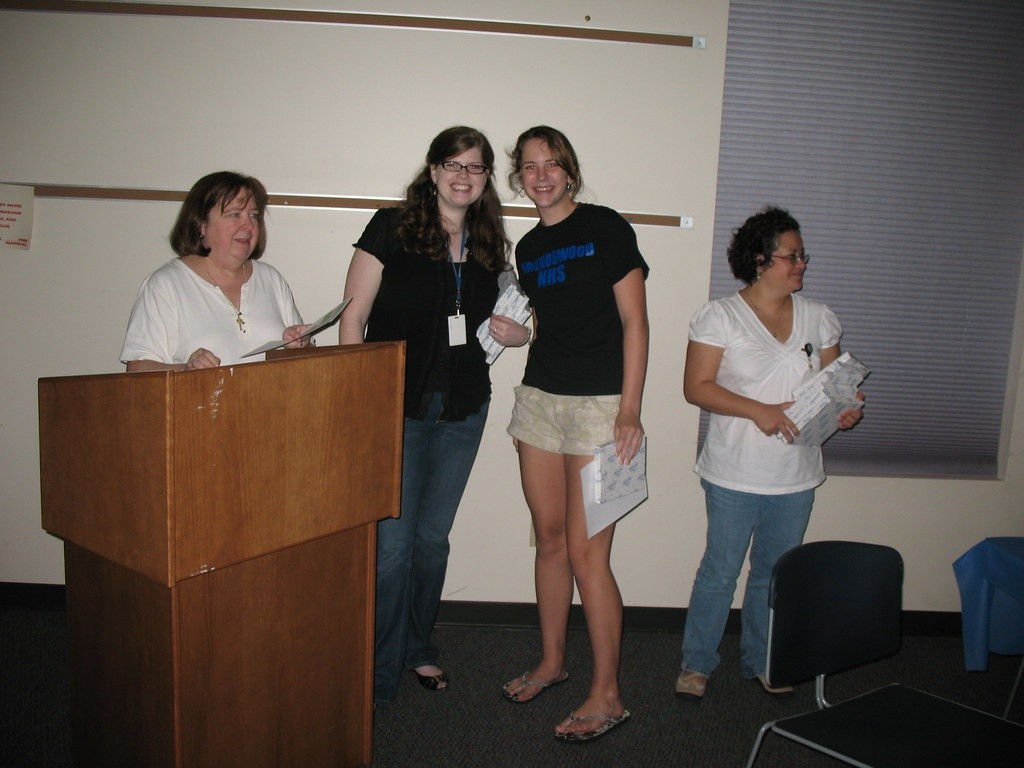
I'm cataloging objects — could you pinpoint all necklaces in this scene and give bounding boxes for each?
[746,289,786,338]
[199,255,245,331]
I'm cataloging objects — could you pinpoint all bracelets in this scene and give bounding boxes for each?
[515,326,531,346]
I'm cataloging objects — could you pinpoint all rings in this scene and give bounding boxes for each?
[494,328,497,334]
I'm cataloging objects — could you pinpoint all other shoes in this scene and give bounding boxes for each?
[757,670,794,693]
[676,667,708,697]
[413,664,449,690]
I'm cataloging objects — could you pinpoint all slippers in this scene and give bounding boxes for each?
[502,665,569,703]
[554,706,631,740]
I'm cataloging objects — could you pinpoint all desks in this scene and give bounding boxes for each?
[951,536,1024,720]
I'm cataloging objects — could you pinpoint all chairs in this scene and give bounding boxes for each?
[745,540,1024,768]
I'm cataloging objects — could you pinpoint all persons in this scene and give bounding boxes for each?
[677,205,864,697]
[507,125,650,743]
[119,172,316,374]
[338,126,537,713]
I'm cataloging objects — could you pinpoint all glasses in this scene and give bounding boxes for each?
[768,253,810,265]
[438,161,488,174]
